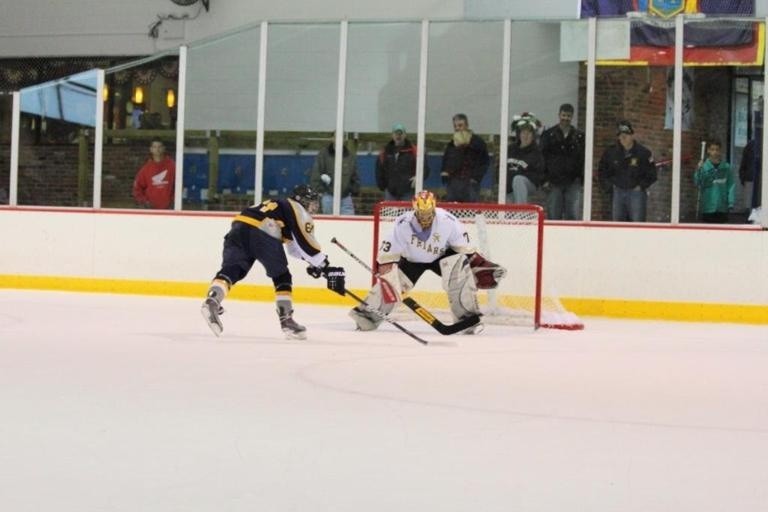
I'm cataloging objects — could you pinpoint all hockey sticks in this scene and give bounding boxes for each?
[345,289,454,348]
[331,237,480,335]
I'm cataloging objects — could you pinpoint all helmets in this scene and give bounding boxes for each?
[290,184,321,212]
[411,190,437,229]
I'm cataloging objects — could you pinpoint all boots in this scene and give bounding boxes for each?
[279,306,306,332]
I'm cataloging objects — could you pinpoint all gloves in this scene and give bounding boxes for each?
[324,267,345,296]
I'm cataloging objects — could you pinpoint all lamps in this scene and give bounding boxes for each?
[133,84,145,109]
[165,86,176,113]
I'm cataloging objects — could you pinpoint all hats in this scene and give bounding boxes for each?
[394,124,406,134]
[616,120,633,136]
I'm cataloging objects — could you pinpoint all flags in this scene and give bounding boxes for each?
[579,0,765,75]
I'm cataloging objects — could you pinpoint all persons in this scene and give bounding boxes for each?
[597,120,657,222]
[309,128,361,216]
[201,183,331,336]
[538,101,586,221]
[131,139,175,210]
[508,112,550,204]
[440,112,492,202]
[374,120,431,200]
[693,139,738,223]
[348,190,505,334]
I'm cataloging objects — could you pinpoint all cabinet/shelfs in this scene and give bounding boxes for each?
[162,145,496,198]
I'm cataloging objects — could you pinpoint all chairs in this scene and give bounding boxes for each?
[208,189,520,215]
